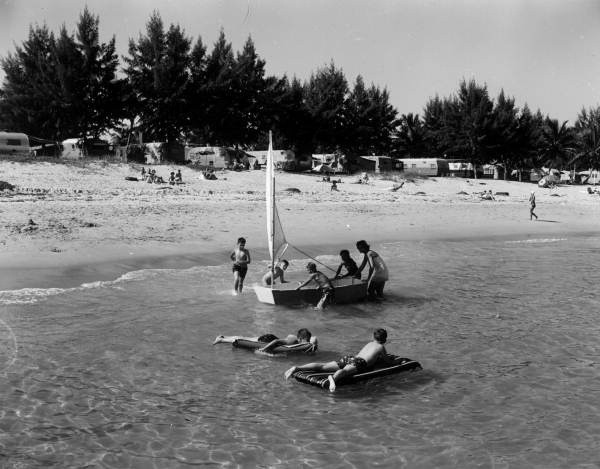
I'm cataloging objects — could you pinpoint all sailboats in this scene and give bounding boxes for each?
[252,130,368,307]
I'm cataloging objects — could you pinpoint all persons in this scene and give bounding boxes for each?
[528,191,537,221]
[284,328,388,393]
[152,170,168,183]
[354,240,389,298]
[363,174,368,183]
[168,172,177,185]
[477,192,495,200]
[144,168,156,184]
[296,262,337,310]
[175,170,182,182]
[322,171,330,182]
[204,161,215,176]
[262,260,291,286]
[334,250,361,280]
[331,181,337,191]
[212,328,311,352]
[230,237,250,293]
